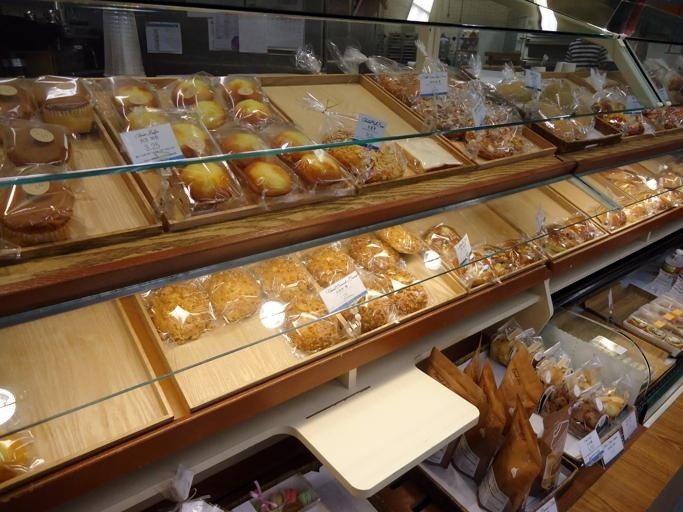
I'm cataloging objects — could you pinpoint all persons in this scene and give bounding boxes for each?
[447,37,458,65]
[382,35,389,60]
[439,33,450,59]
[563,36,608,71]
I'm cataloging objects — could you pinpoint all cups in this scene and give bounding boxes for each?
[102,8,147,78]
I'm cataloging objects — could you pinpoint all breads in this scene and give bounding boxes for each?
[0,68,683,512]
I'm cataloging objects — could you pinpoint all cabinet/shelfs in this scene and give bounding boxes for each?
[0,0,683,512]
